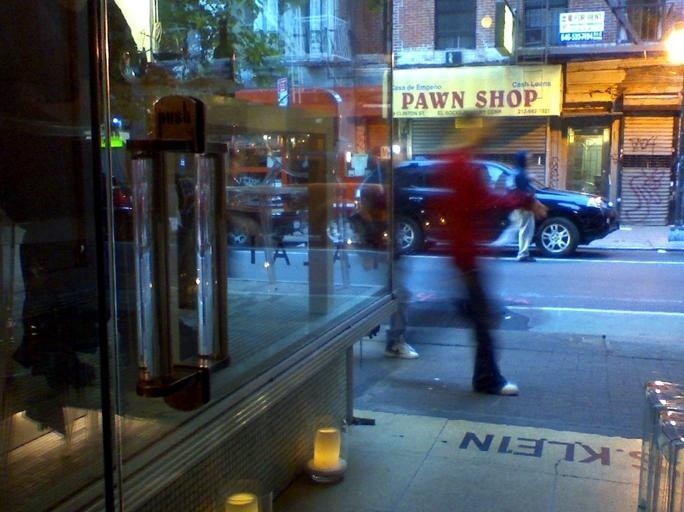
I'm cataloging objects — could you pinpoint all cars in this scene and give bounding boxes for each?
[348,158,622,259]
[223,143,356,247]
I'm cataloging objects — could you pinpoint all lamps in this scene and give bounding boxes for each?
[304,426,349,486]
[224,493,259,512]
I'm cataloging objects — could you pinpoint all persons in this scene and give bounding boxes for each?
[357,135,420,359]
[512,147,539,263]
[107,128,382,320]
[421,106,550,397]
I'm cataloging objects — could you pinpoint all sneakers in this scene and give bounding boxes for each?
[383,342,419,359]
[496,383,520,395]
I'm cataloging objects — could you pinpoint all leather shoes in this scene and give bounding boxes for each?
[517,255,536,262]
